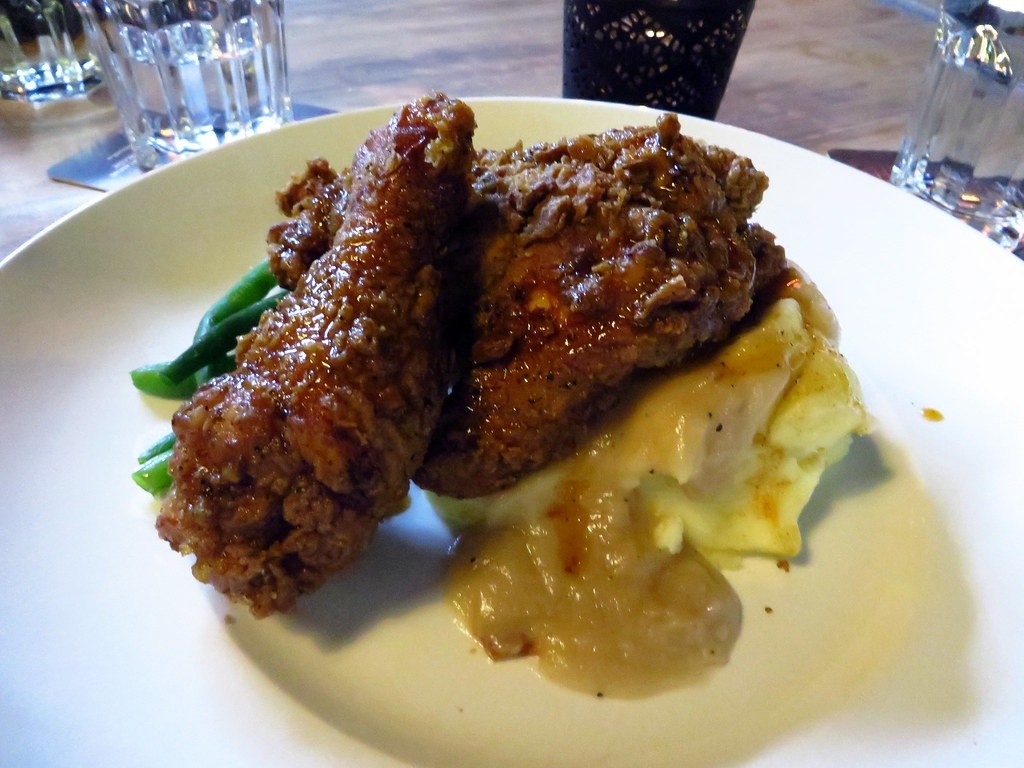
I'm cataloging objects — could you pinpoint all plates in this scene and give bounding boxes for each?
[0,99,1023,767]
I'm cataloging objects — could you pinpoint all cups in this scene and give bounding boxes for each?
[890,0,1024,253]
[0,0,107,104]
[73,0,293,172]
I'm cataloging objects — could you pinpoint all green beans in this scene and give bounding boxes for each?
[131,257,293,498]
